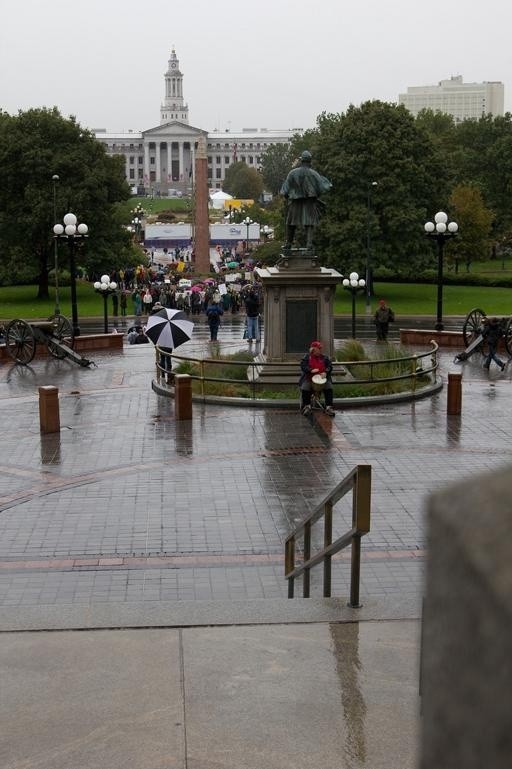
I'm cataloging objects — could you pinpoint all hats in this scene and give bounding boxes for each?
[312,342,321,347]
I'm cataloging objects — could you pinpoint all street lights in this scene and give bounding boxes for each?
[129,202,145,242]
[342,270,367,340]
[367,181,380,297]
[243,217,252,251]
[52,212,90,335]
[50,173,61,315]
[93,273,118,334]
[424,209,460,328]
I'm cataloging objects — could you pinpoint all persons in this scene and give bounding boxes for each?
[374,300,394,344]
[300,342,336,417]
[158,347,173,380]
[481,318,505,371]
[280,151,333,250]
[115,239,264,344]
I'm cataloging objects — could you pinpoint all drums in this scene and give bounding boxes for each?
[311,374,328,391]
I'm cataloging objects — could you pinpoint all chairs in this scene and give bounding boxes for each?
[299,371,324,414]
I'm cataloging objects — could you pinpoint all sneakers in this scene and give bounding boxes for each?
[303,407,312,416]
[326,406,336,416]
